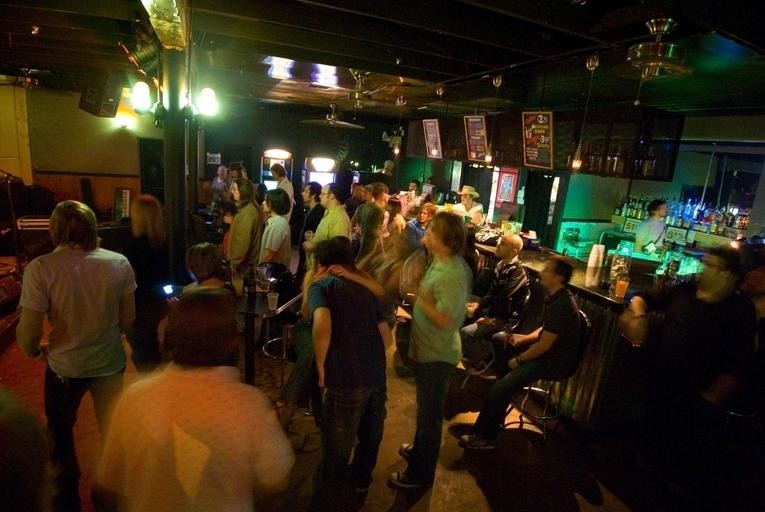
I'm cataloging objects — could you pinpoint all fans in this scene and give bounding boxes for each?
[329,67,405,109]
[300,104,367,130]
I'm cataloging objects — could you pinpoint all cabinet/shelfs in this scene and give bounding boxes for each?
[573,108,686,183]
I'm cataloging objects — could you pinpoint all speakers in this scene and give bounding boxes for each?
[139,135,164,207]
[79,67,125,117]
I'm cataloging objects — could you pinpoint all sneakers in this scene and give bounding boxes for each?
[400,441,413,457]
[467,352,496,376]
[351,480,369,493]
[460,434,495,451]
[460,353,469,362]
[389,470,435,490]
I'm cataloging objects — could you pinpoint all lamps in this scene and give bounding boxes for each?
[693,143,717,219]
[184,87,220,121]
[484,75,502,163]
[430,87,444,156]
[571,55,602,172]
[392,94,406,154]
[129,82,166,127]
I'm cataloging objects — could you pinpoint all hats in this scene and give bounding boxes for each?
[456,184,480,199]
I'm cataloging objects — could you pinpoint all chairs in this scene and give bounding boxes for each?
[455,287,536,395]
[499,308,594,453]
[261,291,312,415]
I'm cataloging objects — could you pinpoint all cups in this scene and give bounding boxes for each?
[266,292,279,311]
[588,243,606,268]
[614,275,632,299]
[585,269,601,287]
[305,230,313,240]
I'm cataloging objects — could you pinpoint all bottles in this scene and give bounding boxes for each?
[610,240,635,282]
[612,193,750,236]
[565,139,657,179]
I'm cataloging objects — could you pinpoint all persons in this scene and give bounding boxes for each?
[16,158,765,512]
